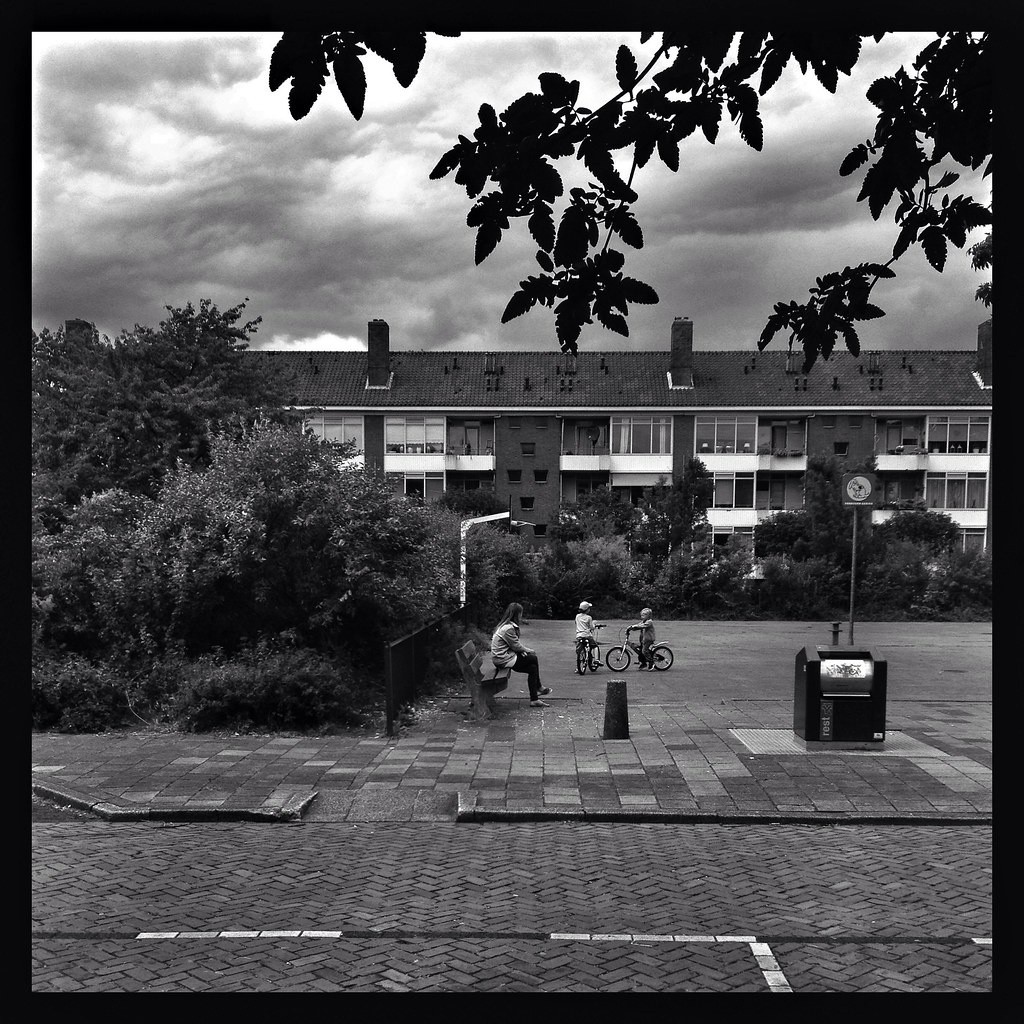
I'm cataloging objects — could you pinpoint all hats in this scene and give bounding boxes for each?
[579,601,592,613]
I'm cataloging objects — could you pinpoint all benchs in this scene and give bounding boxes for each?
[455,640,512,721]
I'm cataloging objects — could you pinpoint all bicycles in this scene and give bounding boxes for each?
[577,623,607,676]
[605,625,674,672]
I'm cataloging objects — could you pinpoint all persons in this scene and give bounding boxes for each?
[391,444,443,454]
[465,443,471,455]
[491,603,553,707]
[450,446,455,455]
[574,601,604,675]
[627,608,656,672]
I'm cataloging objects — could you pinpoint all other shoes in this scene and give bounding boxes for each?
[575,668,579,674]
[639,664,647,669]
[594,659,604,666]
[648,661,656,671]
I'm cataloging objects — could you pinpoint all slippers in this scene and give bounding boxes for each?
[536,685,553,696]
[530,699,550,707]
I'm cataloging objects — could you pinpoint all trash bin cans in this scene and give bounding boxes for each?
[791,643,889,743]
